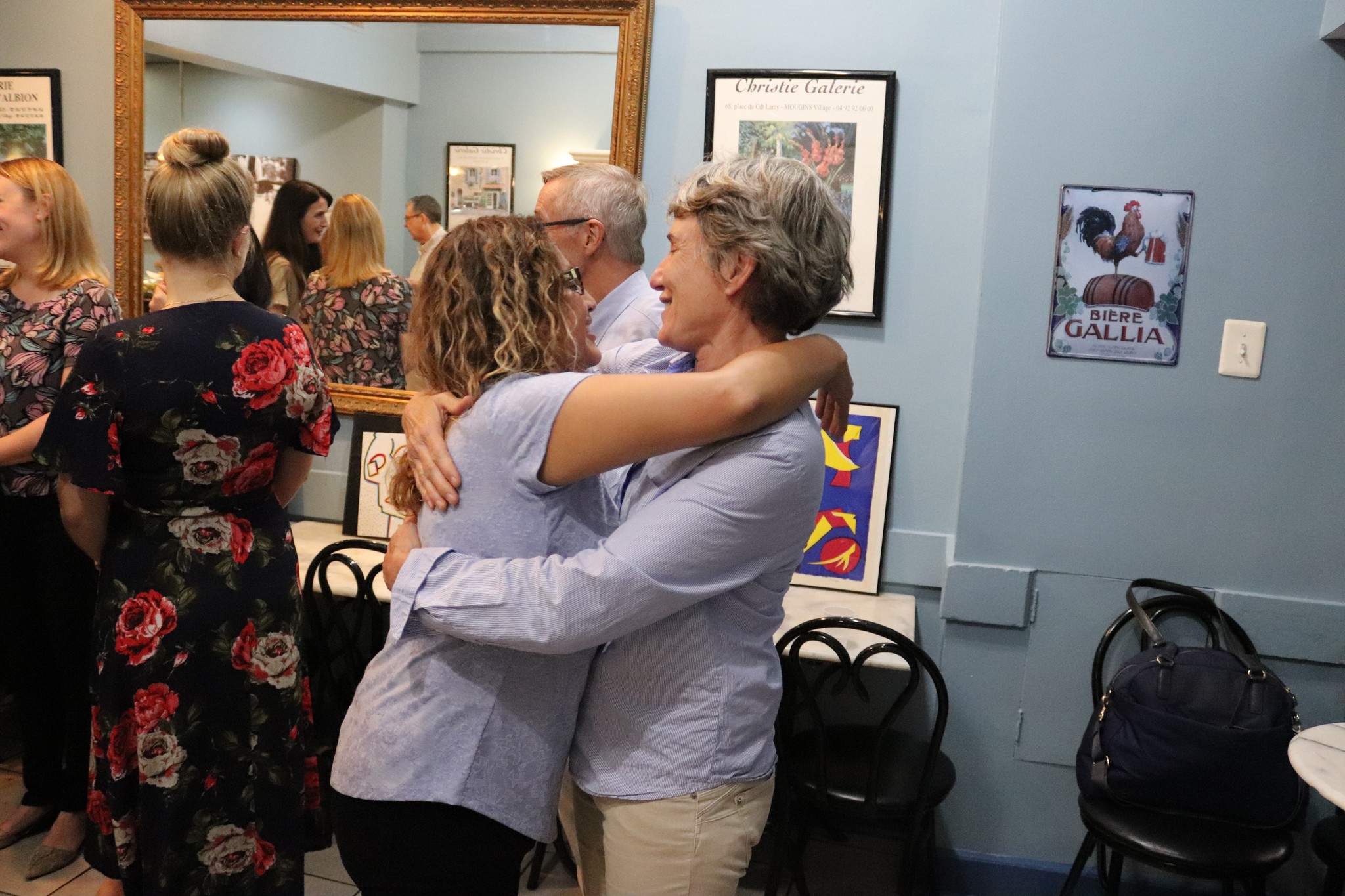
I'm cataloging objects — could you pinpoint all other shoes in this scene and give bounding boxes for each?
[25,834,88,881]
[0,808,56,851]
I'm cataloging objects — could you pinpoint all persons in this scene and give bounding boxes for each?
[533,168,687,373]
[149,179,451,389]
[29,126,334,895]
[326,218,853,895]
[0,158,124,878]
[381,153,856,895]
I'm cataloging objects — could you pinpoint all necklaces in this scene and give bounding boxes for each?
[161,292,238,308]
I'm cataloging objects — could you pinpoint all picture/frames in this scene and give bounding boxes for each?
[788,398,899,596]
[704,69,897,320]
[340,412,407,541]
[0,68,64,167]
[445,142,516,231]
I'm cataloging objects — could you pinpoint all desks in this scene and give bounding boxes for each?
[1287,723,1345,896]
[291,520,915,670]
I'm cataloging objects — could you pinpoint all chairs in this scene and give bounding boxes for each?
[764,618,956,896]
[1063,594,1294,896]
[302,540,386,745]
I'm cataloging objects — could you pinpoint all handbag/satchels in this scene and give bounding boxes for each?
[1083,576,1303,847]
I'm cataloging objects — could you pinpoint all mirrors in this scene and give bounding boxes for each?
[113,0,655,413]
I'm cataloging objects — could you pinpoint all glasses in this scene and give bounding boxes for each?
[557,266,586,297]
[404,214,420,222]
